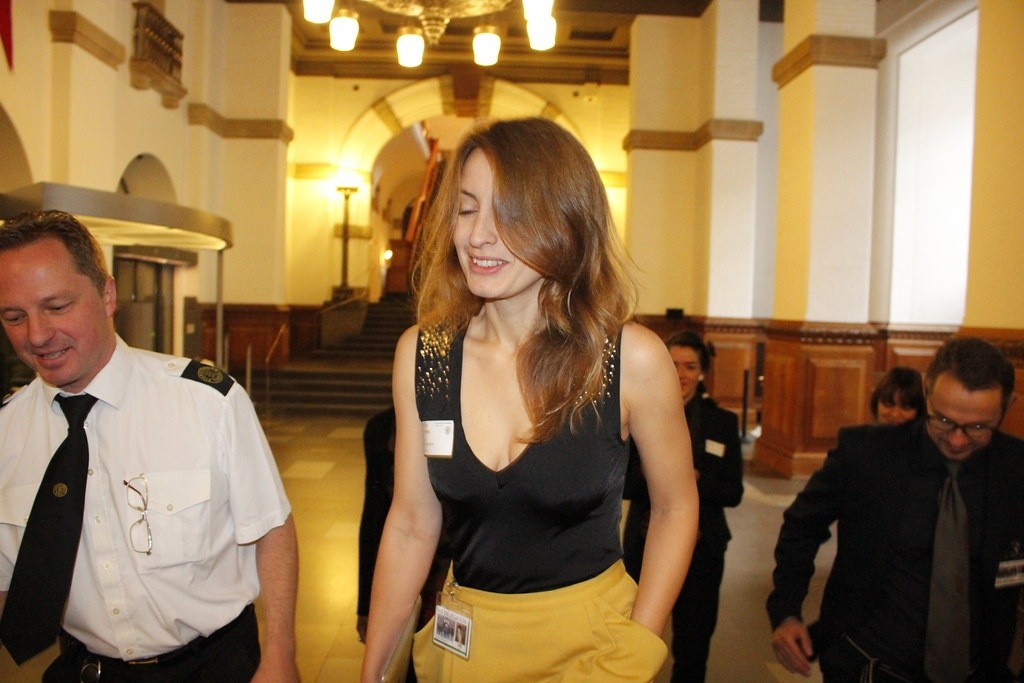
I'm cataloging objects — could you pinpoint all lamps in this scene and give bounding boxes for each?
[333,157,358,295]
[302,0,557,68]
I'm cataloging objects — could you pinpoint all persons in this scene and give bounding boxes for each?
[355,409,450,683]
[620,330,745,683]
[0,208,306,683]
[440,620,454,638]
[765,336,1024,683]
[871,365,925,427]
[455,624,467,644]
[356,120,699,683]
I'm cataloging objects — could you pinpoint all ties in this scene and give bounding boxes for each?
[923,460,971,683]
[1,393,101,667]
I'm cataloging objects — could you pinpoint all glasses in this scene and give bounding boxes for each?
[919,400,1005,436]
[123,474,152,560]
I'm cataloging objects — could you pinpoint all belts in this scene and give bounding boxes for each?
[81,617,242,669]
